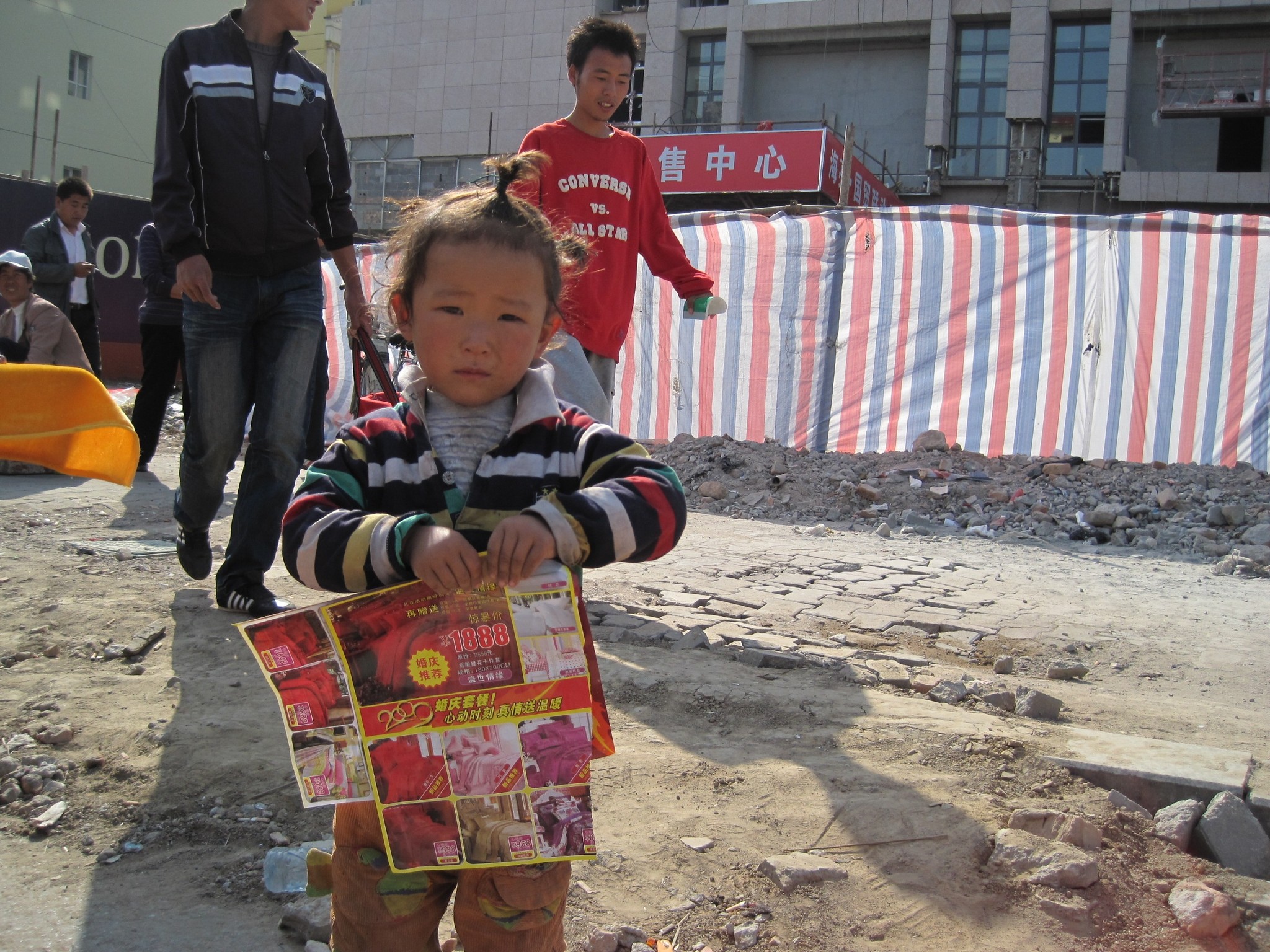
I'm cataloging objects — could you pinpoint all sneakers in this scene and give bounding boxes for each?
[213,569,296,617]
[173,485,212,580]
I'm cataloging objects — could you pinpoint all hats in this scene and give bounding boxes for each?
[0,250,33,274]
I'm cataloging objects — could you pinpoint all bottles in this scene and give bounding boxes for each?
[263,845,333,893]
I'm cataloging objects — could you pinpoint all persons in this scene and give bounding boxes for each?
[0,249,96,376]
[130,224,191,472]
[153,0,378,616]
[281,155,687,952]
[22,180,103,384]
[504,16,714,427]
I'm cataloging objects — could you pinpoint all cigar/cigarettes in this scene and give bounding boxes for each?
[93,267,101,272]
